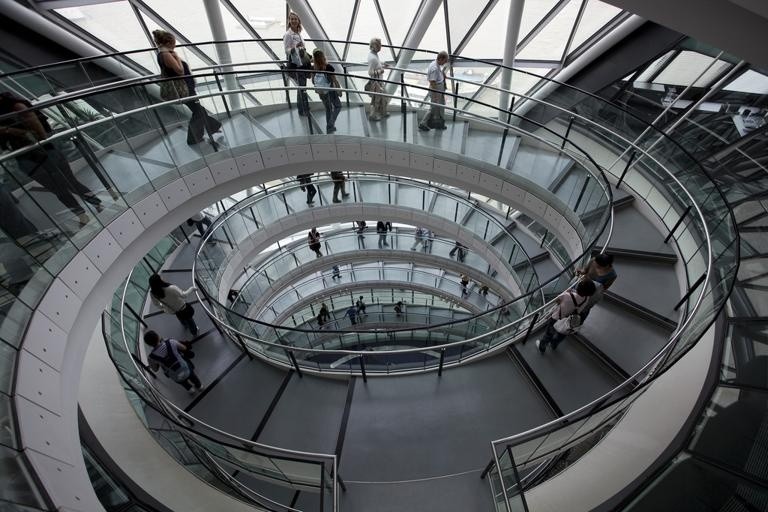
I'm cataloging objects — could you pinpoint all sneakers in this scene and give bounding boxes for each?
[536,340,541,349]
[187,385,204,394]
[326,126,336,134]
[333,193,349,202]
[418,124,429,131]
[369,112,390,121]
[195,326,199,337]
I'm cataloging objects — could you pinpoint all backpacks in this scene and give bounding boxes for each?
[149,338,192,383]
[553,292,587,335]
[313,73,332,95]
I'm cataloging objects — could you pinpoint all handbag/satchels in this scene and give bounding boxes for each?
[160,73,190,104]
[187,218,195,226]
[428,118,444,128]
[364,79,385,96]
[174,303,195,324]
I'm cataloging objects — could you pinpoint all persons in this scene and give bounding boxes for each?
[317,303,330,327]
[418,50,448,132]
[535,279,596,355]
[411,226,469,264]
[0,91,105,227]
[1,183,61,257]
[344,296,368,325]
[366,38,391,121]
[152,29,223,146]
[228,289,241,305]
[574,254,617,325]
[394,302,403,317]
[460,272,489,298]
[297,173,318,208]
[330,171,350,203]
[143,329,204,395]
[357,220,392,249]
[311,48,343,131]
[148,273,198,337]
[283,11,312,117]
[308,226,323,258]
[332,265,342,280]
[191,211,216,245]
